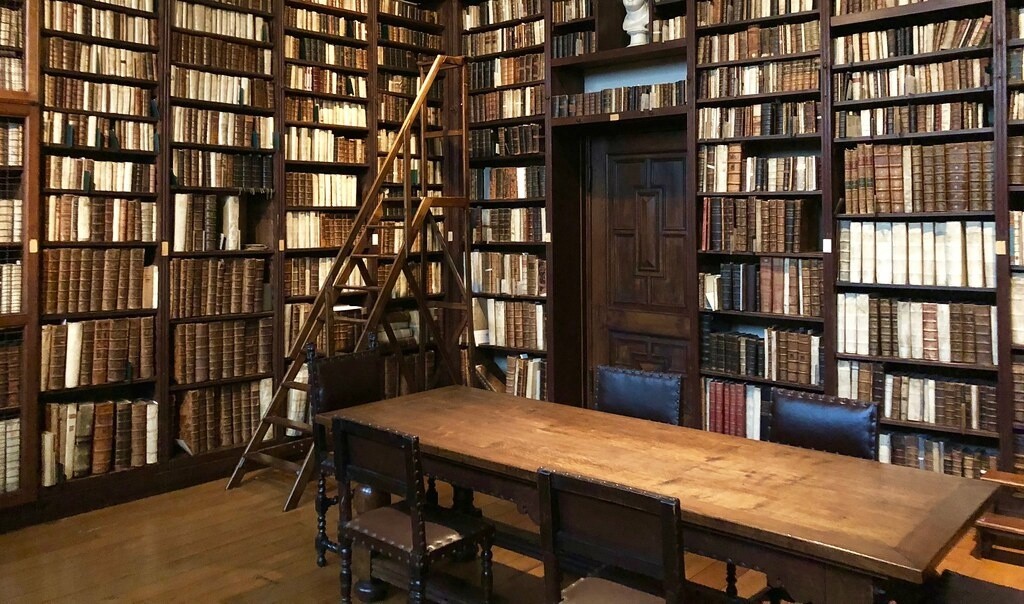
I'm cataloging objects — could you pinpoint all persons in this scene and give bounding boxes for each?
[622,0,649,48]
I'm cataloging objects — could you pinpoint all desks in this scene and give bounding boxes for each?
[317,384,1001,604]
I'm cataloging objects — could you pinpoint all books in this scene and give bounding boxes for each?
[550,80,687,119]
[652,16,688,43]
[551,0,596,59]
[692,0,1024,479]
[0,0,547,493]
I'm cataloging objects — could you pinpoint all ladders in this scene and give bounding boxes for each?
[223,51,478,513]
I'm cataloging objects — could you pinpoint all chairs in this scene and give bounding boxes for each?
[537,467,752,604]
[769,386,879,464]
[331,414,495,604]
[307,332,386,565]
[595,366,683,427]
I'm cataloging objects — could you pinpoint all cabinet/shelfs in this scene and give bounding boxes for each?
[0,0,1024,529]
